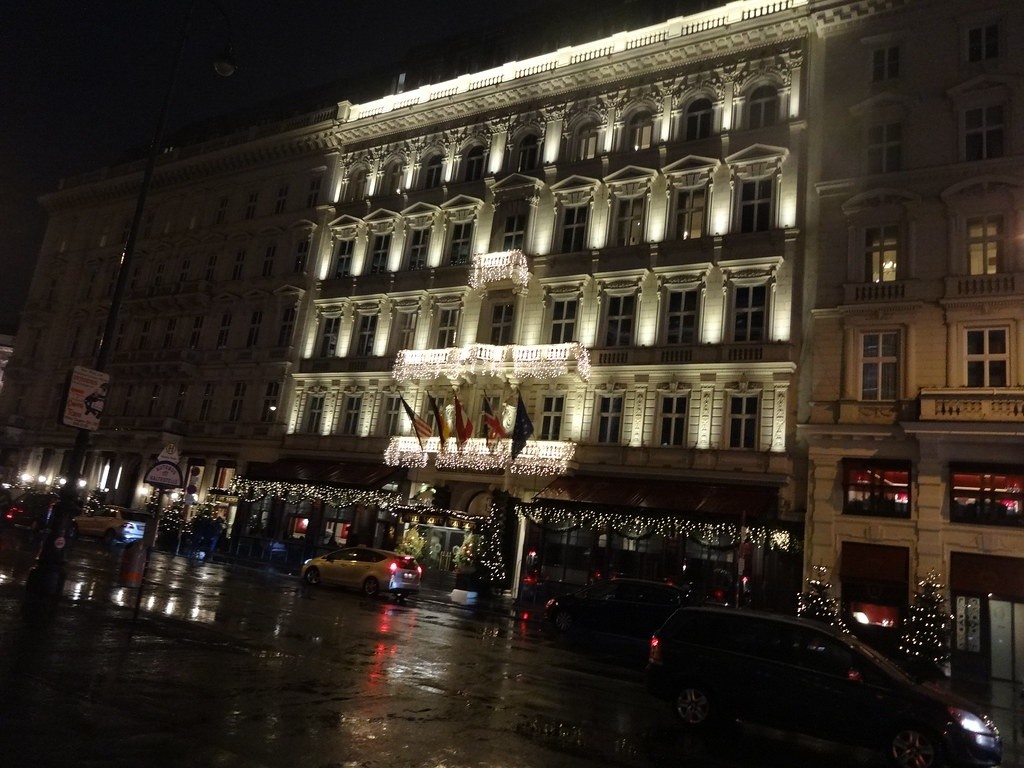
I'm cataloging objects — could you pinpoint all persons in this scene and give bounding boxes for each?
[183,510,229,563]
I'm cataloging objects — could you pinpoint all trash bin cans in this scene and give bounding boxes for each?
[116,540,147,588]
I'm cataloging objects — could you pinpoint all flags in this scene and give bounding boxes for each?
[429,396,449,448]
[482,396,505,453]
[511,394,534,461]
[401,396,432,439]
[453,396,473,444]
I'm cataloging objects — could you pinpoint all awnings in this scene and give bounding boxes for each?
[531,476,773,517]
[245,455,401,491]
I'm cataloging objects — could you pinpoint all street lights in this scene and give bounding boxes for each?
[22,0,239,619]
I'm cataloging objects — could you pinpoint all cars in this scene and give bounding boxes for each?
[640,605,1003,768]
[5,491,59,533]
[543,578,703,667]
[300,546,423,602]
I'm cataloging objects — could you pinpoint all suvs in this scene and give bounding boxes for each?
[69,505,160,548]
